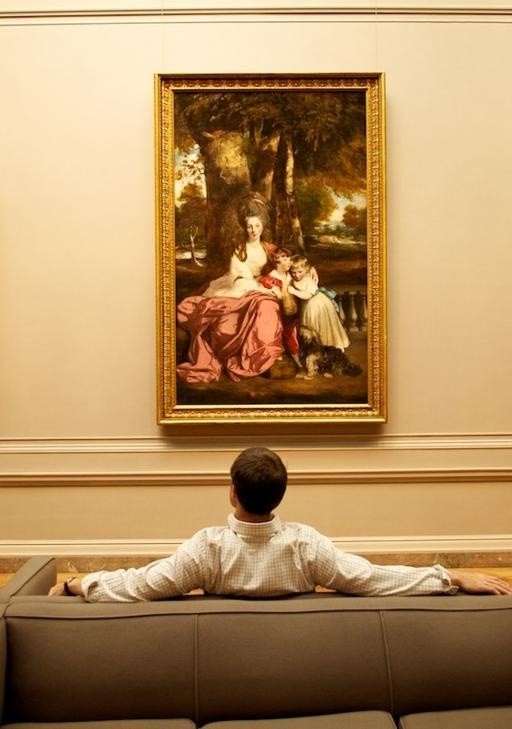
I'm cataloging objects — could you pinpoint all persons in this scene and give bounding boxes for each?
[177,190,351,393]
[43,446,511,599]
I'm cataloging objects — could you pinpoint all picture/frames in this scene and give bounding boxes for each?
[153,71,390,428]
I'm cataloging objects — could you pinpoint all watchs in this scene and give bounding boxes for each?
[64,576,78,596]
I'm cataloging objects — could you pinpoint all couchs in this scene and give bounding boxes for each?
[1,552,510,729]
